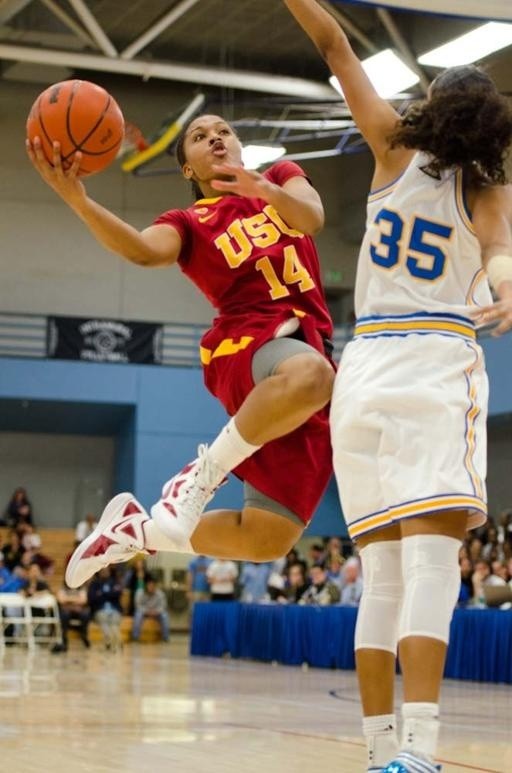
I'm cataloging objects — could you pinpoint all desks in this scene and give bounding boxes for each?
[193,600,511,686]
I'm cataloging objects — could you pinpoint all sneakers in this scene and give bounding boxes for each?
[368,751,443,773]
[65,491,157,589]
[150,456,228,544]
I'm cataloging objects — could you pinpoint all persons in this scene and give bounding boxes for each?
[282,0,512,773]
[57,512,189,646]
[455,508,511,610]
[1,486,56,646]
[25,111,340,588]
[189,535,364,607]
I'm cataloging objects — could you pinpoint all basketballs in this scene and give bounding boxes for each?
[24,81,124,177]
